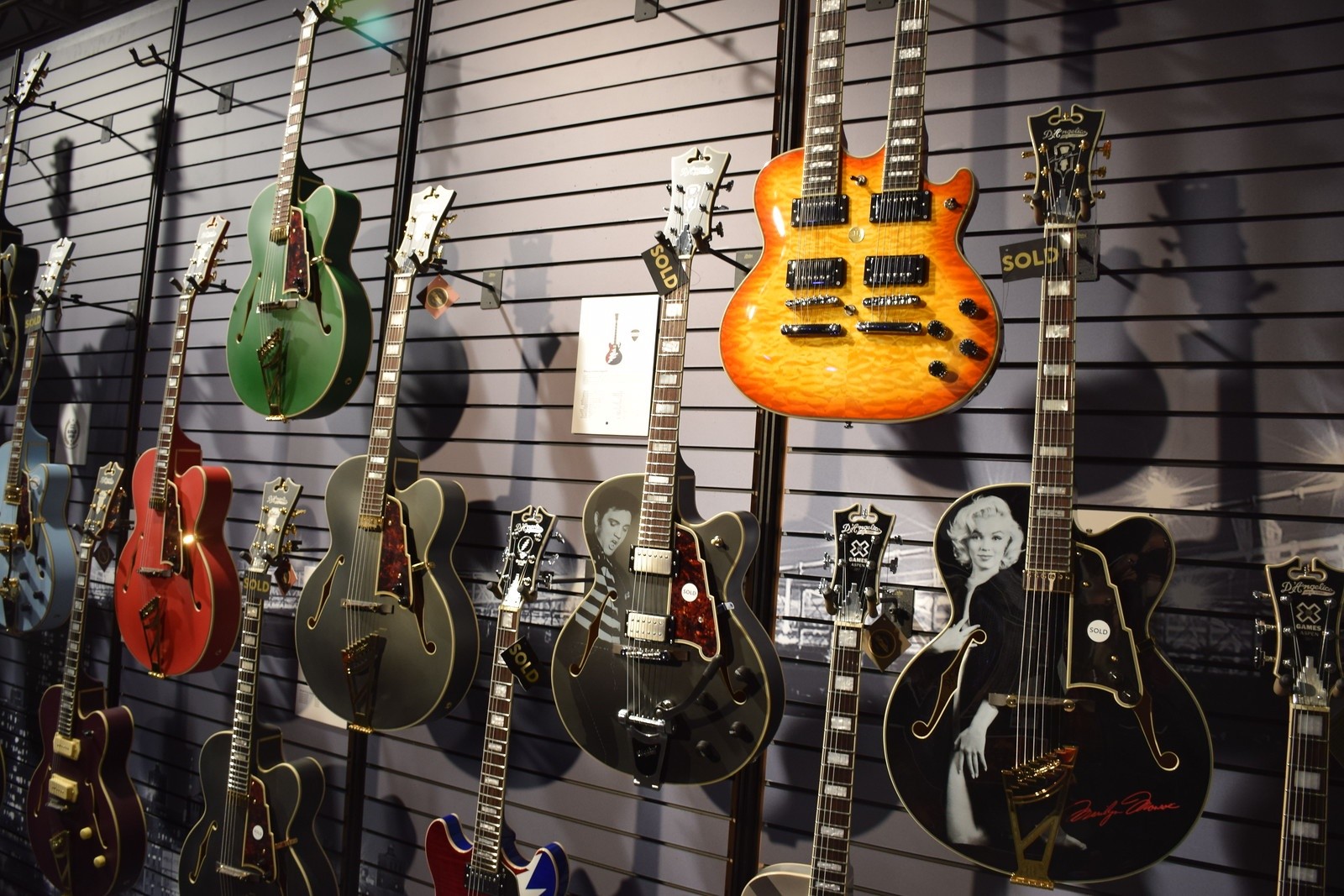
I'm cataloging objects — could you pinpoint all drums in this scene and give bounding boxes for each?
[0,46,54,410]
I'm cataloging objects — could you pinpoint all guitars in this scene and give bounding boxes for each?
[424,505,572,895]
[1246,557,1344,896]
[291,184,486,735]
[739,506,898,895]
[0,232,80,637]
[549,138,792,793]
[715,0,1005,431]
[175,475,343,896]
[21,462,150,894]
[225,0,376,422]
[112,214,242,676]
[880,102,1217,890]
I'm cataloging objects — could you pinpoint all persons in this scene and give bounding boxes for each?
[895,496,1087,851]
[566,486,657,770]
